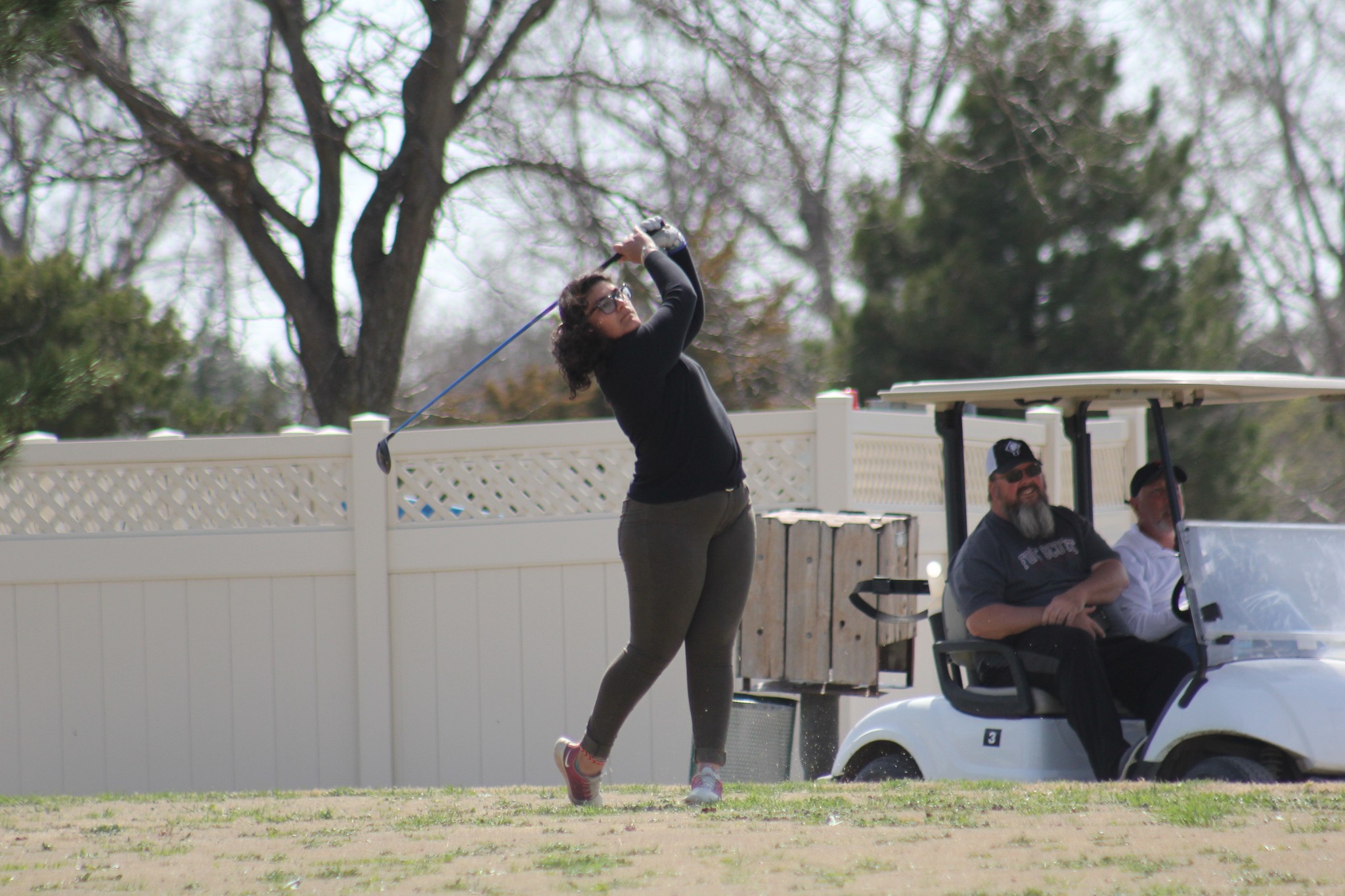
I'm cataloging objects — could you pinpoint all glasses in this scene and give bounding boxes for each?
[586,284,632,318]
[997,463,1041,482]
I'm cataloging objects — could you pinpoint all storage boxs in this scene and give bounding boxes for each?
[734,509,918,694]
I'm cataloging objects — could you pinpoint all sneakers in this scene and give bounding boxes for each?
[553,737,605,808]
[684,765,723,806]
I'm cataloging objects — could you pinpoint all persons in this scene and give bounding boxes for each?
[946,439,1176,782]
[1104,460,1322,668]
[554,218,757,813]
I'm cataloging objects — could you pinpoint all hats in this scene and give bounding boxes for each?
[1131,462,1187,498]
[984,438,1043,475]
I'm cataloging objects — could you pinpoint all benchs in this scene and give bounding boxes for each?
[929,580,1141,720]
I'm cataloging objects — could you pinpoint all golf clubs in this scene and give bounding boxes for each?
[375,216,666,476]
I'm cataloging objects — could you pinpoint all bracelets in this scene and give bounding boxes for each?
[641,244,656,266]
[671,230,685,248]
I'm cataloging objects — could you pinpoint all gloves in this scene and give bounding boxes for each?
[639,216,688,253]
